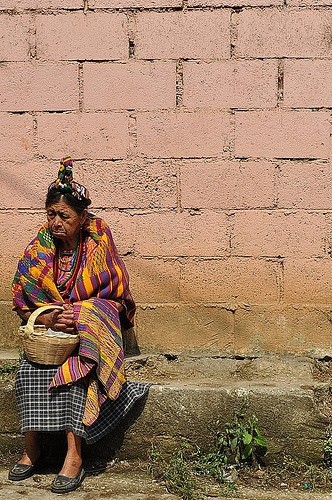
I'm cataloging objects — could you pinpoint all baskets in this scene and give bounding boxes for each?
[17,305,80,365]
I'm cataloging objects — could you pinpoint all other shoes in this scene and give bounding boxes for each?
[8,448,43,481]
[50,460,86,493]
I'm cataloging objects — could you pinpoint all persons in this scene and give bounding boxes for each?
[8,155,153,493]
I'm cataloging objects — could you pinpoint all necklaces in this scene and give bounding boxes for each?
[55,228,83,298]
